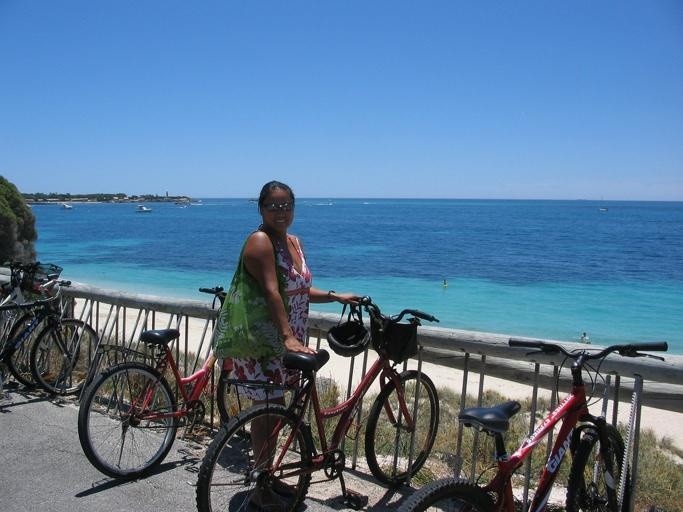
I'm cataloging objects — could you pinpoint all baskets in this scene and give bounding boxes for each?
[370,319,419,361]
[22,261,63,296]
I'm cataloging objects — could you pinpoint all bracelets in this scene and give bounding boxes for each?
[327,290,336,302]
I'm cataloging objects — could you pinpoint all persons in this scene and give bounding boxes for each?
[208,180,361,511]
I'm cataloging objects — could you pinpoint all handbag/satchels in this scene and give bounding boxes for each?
[210,230,290,362]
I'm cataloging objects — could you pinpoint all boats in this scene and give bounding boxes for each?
[135,204,152,212]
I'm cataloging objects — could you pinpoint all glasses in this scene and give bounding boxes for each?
[261,202,295,212]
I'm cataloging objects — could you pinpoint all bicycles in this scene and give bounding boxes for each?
[0,261,99,395]
[193,292,439,512]
[78,288,252,478]
[396,338,669,511]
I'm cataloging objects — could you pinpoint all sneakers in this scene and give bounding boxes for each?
[256,472,297,497]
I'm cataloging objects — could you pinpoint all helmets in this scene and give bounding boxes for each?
[326,320,371,358]
